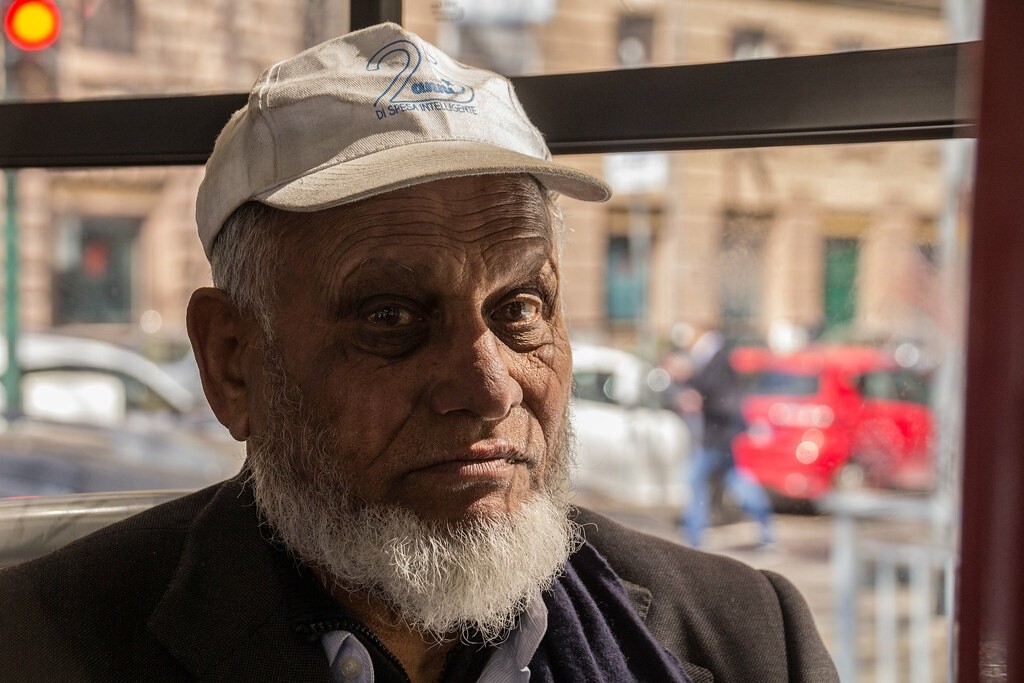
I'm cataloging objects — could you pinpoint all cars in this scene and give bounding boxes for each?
[559,341,696,520]
[0,332,243,499]
[723,345,946,513]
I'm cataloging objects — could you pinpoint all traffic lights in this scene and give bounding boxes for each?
[0,0,58,82]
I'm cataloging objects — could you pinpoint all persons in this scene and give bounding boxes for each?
[1,22,844,683]
[665,310,773,551]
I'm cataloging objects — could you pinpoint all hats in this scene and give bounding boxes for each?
[196,22,613,262]
